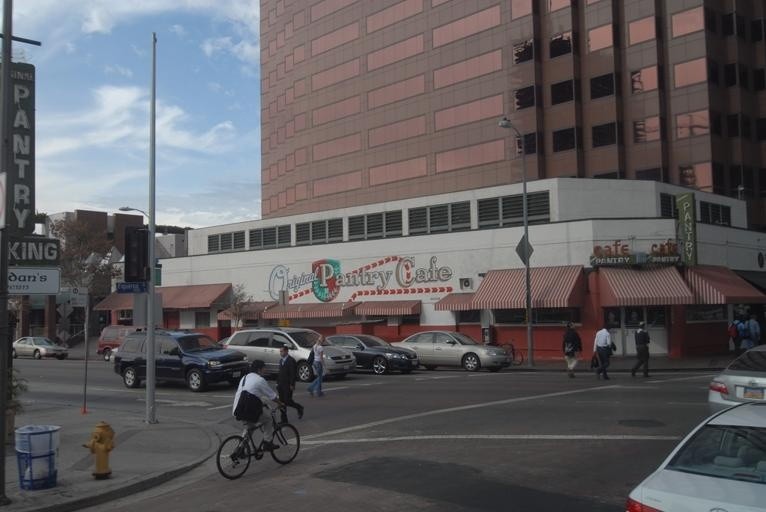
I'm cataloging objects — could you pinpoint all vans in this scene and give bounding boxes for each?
[113,328,248,392]
[228,327,356,380]
[94,325,146,361]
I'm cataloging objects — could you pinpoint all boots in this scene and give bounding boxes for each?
[263,440,280,449]
[234,445,246,458]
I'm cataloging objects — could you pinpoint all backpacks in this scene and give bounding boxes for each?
[728,321,742,337]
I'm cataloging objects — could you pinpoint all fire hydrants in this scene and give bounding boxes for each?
[83,420,116,478]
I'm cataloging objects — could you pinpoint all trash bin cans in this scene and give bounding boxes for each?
[14,424,60,491]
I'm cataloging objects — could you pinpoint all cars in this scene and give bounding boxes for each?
[213,334,230,348]
[625,402,766,512]
[327,333,418,374]
[11,336,68,359]
[707,345,765,415]
[390,330,514,372]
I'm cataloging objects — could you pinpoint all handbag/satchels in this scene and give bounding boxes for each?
[592,356,598,368]
[307,351,314,364]
[234,390,263,422]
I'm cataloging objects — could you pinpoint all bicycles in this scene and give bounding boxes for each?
[498,340,525,366]
[217,403,303,480]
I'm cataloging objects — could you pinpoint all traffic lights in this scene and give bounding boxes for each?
[125,226,144,281]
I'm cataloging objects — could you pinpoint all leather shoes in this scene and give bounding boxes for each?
[297,405,304,419]
[278,419,288,424]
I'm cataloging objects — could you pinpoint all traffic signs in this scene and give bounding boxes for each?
[117,282,148,293]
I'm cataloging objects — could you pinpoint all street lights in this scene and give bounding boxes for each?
[499,117,536,367]
[120,206,152,223]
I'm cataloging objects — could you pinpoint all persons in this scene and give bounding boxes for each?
[632,321,650,377]
[731,316,745,357]
[55,337,63,346]
[594,325,612,380]
[231,360,287,459]
[306,336,326,396]
[275,346,304,425]
[745,314,761,349]
[562,321,582,379]
